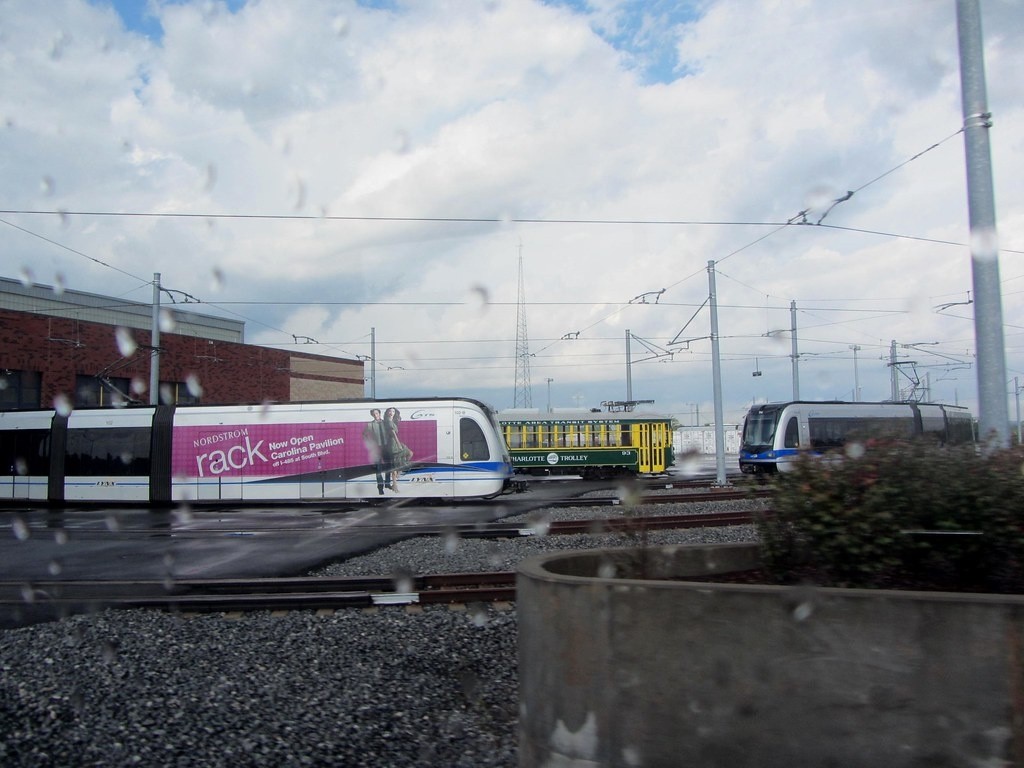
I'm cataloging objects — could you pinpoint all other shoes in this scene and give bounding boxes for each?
[378,488,384,496]
[392,484,400,496]
[385,483,393,490]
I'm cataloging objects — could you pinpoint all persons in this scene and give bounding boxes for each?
[362,408,392,495]
[383,407,413,492]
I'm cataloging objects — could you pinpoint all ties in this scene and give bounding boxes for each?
[377,421,386,448]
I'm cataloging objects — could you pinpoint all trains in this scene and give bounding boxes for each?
[1,394,525,514]
[491,404,681,484]
[737,397,973,482]
